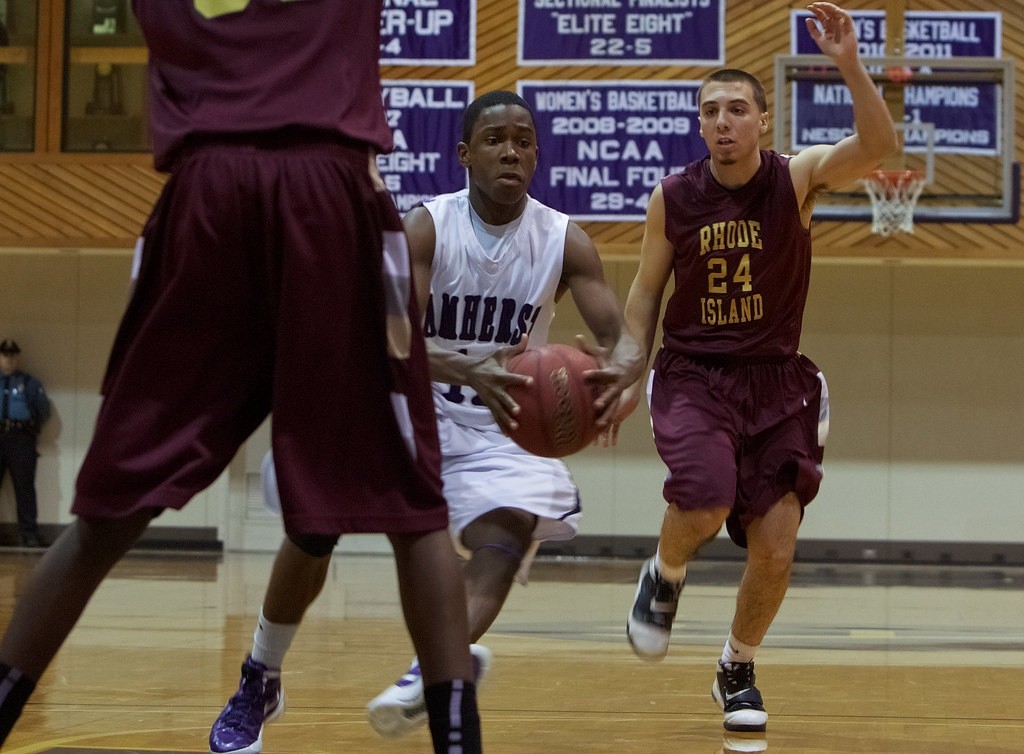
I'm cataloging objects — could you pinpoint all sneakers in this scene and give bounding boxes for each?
[625,556,688,665]
[364,643,492,741]
[0,666,37,747]
[710,656,770,733]
[423,679,484,754]
[208,655,286,754]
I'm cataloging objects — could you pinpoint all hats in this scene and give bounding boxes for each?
[0,338,21,354]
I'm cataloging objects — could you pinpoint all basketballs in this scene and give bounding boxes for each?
[502,343,604,459]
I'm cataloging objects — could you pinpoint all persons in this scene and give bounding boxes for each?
[594,2,900,734]
[0,1,480,754]
[209,91,644,754]
[0,340,49,548]
